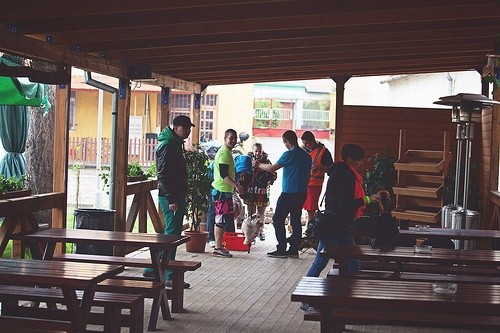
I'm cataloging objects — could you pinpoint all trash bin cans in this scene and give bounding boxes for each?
[75,208,116,257]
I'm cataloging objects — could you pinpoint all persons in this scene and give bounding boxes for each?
[206,155,252,248]
[239,143,278,240]
[211,128,246,258]
[143,115,195,288]
[301,131,332,235]
[252,130,312,258]
[300,144,381,311]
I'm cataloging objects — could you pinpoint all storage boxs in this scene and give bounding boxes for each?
[223,232,249,251]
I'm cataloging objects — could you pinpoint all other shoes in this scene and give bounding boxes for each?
[286,244,299,258]
[266,245,287,257]
[166,279,190,289]
[258,233,266,240]
[208,240,215,248]
[143,270,156,277]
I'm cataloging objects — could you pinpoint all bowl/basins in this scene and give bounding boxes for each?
[432,282,458,293]
[414,225,431,232]
[413,245,433,254]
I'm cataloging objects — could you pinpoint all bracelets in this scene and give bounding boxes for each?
[359,195,371,209]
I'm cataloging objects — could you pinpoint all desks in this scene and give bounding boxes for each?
[291,276,500,333]
[320,245,500,274]
[397,226,500,240]
[9,229,191,332]
[0,259,124,333]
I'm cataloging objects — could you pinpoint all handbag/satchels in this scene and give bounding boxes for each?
[304,210,336,241]
[370,192,400,251]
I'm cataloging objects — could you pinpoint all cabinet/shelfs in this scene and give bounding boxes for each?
[392,129,453,229]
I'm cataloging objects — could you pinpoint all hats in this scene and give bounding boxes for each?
[173,115,195,128]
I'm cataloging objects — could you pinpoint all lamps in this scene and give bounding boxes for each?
[440,93,500,250]
[434,100,494,228]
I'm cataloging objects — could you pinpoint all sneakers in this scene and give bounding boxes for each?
[212,244,233,258]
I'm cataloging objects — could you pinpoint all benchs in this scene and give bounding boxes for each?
[0,285,145,333]
[53,253,201,313]
[304,259,500,333]
[78,279,174,299]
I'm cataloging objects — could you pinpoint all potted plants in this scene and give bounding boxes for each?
[0,173,32,200]
[183,142,214,253]
[96,162,147,195]
